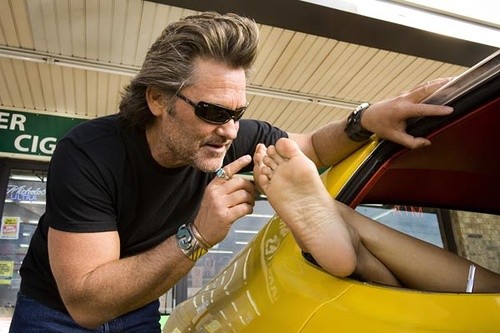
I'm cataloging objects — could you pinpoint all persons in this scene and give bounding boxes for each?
[9,11,457,333]
[253,137,500,293]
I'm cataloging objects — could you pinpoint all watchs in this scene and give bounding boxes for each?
[344,102,375,142]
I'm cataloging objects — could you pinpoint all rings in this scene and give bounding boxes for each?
[216,168,231,181]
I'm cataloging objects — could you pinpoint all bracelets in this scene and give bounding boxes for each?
[191,224,213,249]
[176,222,208,262]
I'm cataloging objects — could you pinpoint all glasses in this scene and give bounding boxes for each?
[173,91,245,125]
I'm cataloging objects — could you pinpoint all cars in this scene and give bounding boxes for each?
[161,48,500,332]
